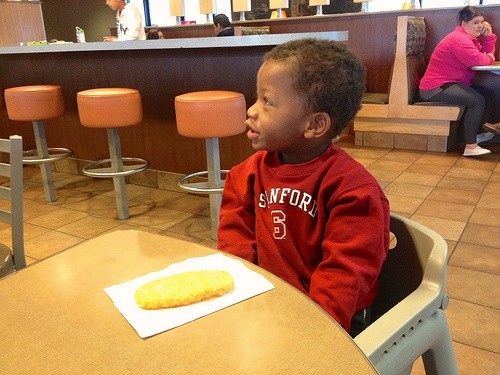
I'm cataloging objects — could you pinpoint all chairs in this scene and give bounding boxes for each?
[0,136,25,277]
[351,212,459,375]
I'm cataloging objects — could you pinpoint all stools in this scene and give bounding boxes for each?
[77,88,148,220]
[3,85,74,203]
[176,91,247,240]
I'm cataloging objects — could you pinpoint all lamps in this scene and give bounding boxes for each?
[170,0,370,25]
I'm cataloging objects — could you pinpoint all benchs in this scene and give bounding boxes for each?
[234,25,272,36]
[353,15,464,154]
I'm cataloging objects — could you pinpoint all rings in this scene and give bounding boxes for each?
[488,25,492,27]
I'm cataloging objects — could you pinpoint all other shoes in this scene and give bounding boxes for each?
[477,133,494,143]
[463,146,491,156]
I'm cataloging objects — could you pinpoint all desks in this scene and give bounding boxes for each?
[471,61,500,76]
[0,230,379,375]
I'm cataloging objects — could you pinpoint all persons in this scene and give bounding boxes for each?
[418,5,498,156]
[102,0,146,42]
[147,28,166,40]
[213,14,234,36]
[217,37,391,341]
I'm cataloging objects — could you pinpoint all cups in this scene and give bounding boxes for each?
[75,30,85,43]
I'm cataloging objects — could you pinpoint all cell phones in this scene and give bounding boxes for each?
[481,28,485,34]
